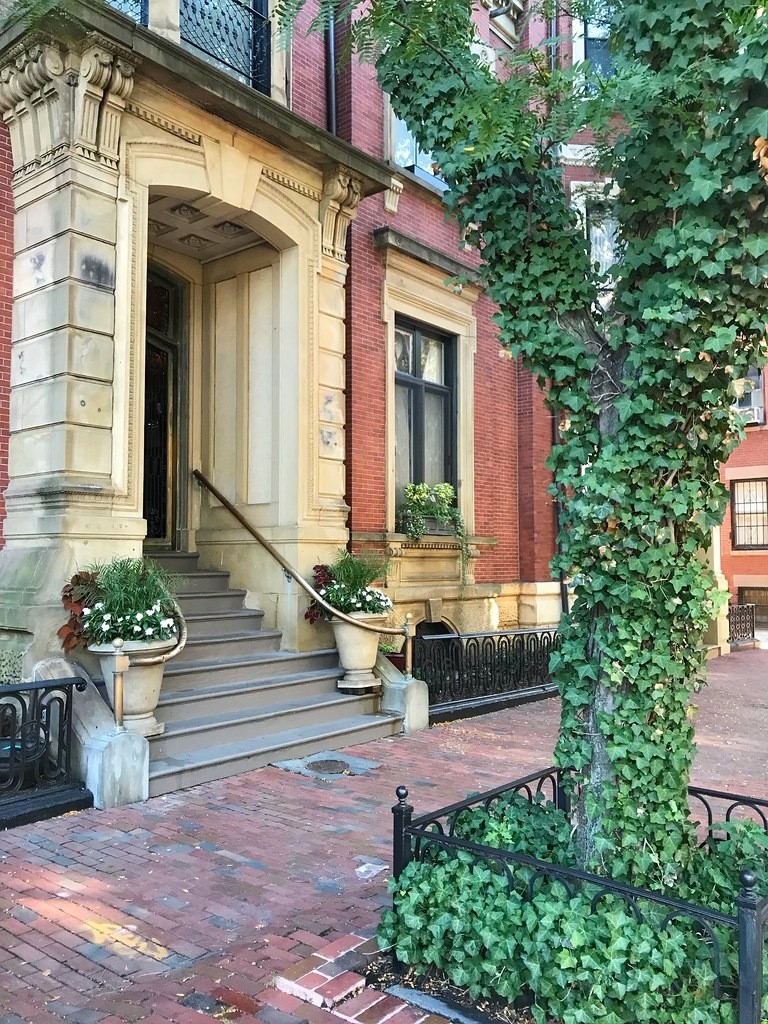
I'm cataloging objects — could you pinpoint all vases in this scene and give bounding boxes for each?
[87,638,179,736]
[401,515,457,536]
[323,611,389,688]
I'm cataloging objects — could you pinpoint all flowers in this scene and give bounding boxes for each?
[56,552,189,658]
[304,546,395,625]
[395,482,468,628]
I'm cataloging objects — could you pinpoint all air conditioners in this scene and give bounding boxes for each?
[741,407,761,425]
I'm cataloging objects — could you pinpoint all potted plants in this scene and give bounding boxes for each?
[376,635,405,673]
